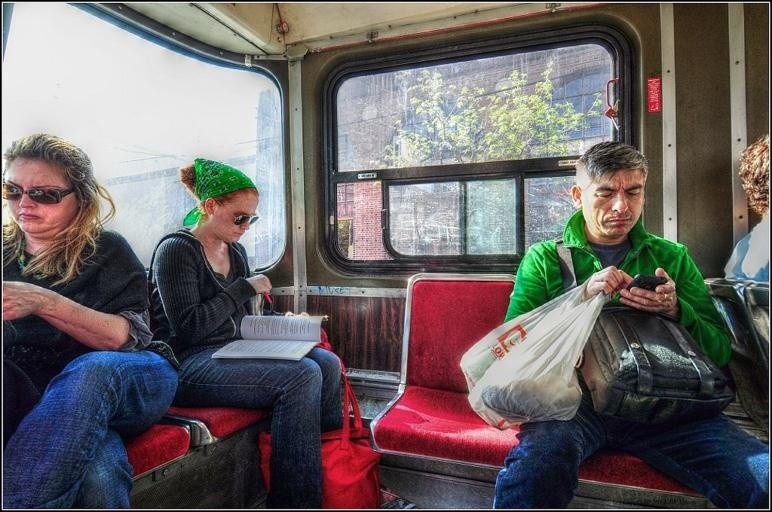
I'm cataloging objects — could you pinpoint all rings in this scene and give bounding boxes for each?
[657,294,667,302]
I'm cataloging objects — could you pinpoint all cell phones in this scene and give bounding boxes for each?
[618,274,667,301]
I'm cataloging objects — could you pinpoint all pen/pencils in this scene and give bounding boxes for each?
[264,292,272,304]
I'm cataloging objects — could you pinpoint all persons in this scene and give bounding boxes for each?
[3,133,182,509]
[491,141,770,509]
[723,135,770,282]
[146,156,340,508]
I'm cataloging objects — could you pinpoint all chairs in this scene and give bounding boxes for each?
[367,266,769,508]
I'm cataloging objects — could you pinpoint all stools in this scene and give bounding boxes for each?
[137,316,336,447]
[117,425,199,482]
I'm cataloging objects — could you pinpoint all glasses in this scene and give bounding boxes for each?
[2,182,74,204]
[213,197,259,225]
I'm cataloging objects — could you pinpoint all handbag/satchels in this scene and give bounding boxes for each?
[579,305,734,421]
[260,427,382,508]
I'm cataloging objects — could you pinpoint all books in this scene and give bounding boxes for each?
[213,314,330,362]
